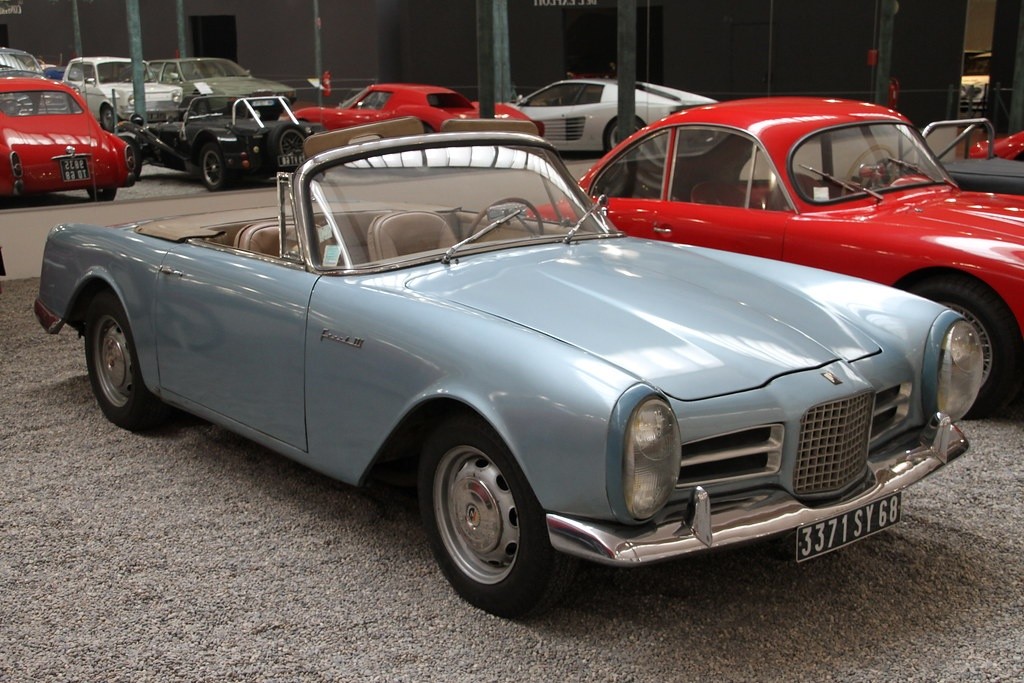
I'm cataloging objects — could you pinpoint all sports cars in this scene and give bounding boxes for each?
[285,84,547,159]
[116,90,329,196]
[31,127,980,616]
[518,95,1024,422]
[147,56,297,124]
[514,76,723,159]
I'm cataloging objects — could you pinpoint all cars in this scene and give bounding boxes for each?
[961,50,993,111]
[60,55,185,134]
[1,45,46,78]
[969,130,1024,163]
[1,77,136,204]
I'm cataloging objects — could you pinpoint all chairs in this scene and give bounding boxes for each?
[235,219,301,258]
[694,172,825,212]
[368,210,456,264]
